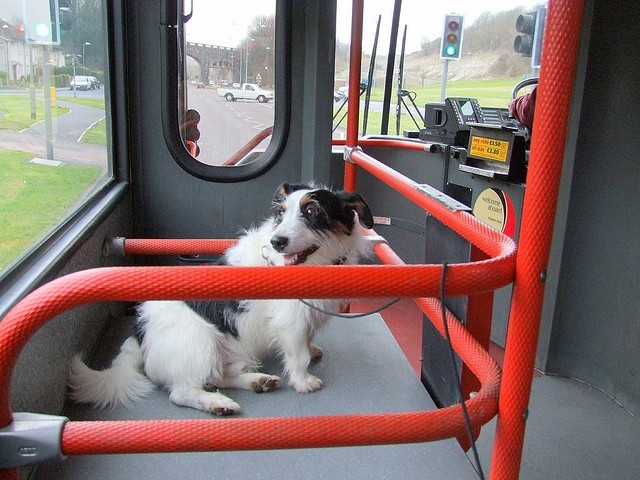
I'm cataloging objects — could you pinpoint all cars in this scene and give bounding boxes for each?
[70,76,91,91]
[197,82,204,88]
[233,83,240,88]
[90,76,100,89]
[221,80,228,86]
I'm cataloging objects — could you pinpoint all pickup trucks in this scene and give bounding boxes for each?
[218,83,273,103]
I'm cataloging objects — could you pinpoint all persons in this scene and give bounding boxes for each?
[508,84,538,129]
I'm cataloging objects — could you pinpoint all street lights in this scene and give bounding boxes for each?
[82,42,91,75]
[245,39,254,82]
[2,25,9,87]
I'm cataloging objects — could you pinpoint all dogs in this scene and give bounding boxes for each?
[64,176,375,419]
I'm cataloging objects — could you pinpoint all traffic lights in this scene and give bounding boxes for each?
[49,0,73,45]
[440,14,464,59]
[514,5,546,68]
